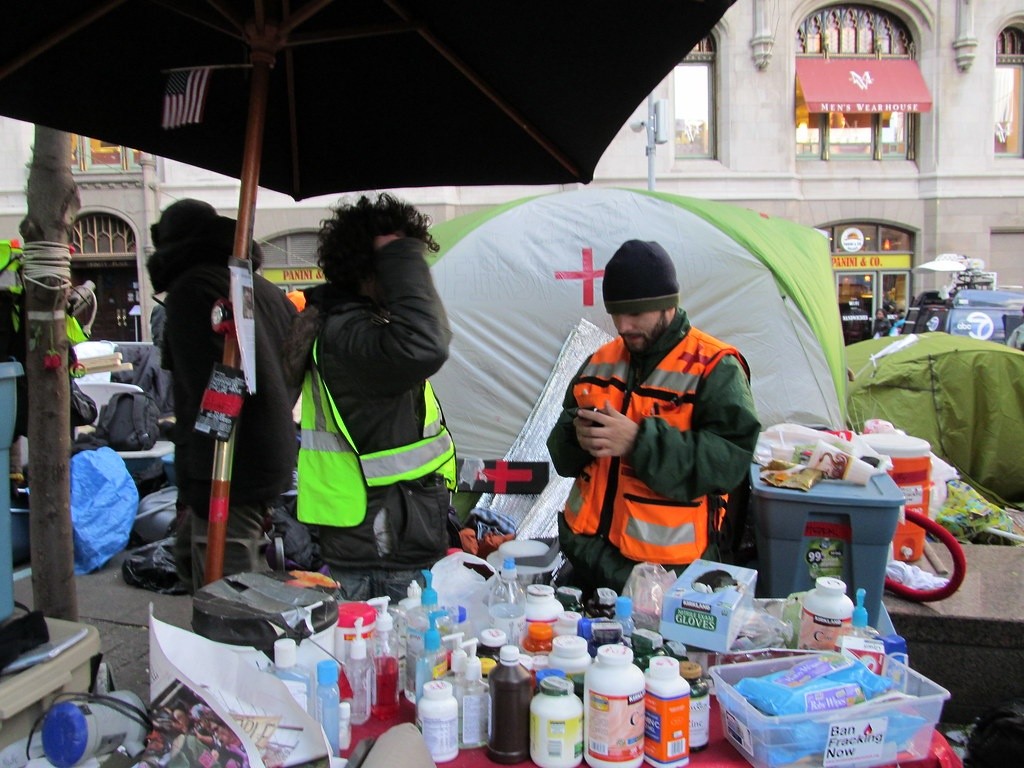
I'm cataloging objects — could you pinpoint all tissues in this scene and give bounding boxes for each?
[658,555,760,655]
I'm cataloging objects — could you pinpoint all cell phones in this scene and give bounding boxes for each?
[581,406,605,427]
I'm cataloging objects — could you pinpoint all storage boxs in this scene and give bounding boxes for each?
[0,607,104,753]
[745,443,907,625]
[706,647,950,768]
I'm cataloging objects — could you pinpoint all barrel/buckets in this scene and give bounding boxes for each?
[859,433,933,563]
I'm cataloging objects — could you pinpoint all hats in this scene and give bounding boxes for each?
[151,199,217,251]
[602,239,680,314]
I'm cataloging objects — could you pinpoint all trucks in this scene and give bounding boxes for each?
[901,288,1024,344]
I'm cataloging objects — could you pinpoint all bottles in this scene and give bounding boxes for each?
[271,547,710,768]
[797,577,855,651]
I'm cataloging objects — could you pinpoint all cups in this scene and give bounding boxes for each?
[807,439,875,486]
[770,444,795,463]
[40,690,148,768]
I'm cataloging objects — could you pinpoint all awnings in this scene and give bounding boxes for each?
[796,57,933,113]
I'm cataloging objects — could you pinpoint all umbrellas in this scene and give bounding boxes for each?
[0,0,732,584]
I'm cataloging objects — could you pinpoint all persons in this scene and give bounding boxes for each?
[132,688,251,768]
[548,240,760,602]
[290,194,458,605]
[874,309,905,336]
[147,198,312,597]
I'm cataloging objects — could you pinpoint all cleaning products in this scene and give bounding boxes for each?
[783,572,911,699]
[269,552,712,768]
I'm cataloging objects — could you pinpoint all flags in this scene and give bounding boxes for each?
[162,67,211,128]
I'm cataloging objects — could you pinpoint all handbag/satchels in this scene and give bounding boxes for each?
[73,381,97,425]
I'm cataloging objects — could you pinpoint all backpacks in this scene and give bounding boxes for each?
[95,391,160,451]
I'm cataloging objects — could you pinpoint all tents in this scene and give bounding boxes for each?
[419,186,848,527]
[846,332,1024,507]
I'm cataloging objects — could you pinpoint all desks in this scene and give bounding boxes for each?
[335,642,964,768]
